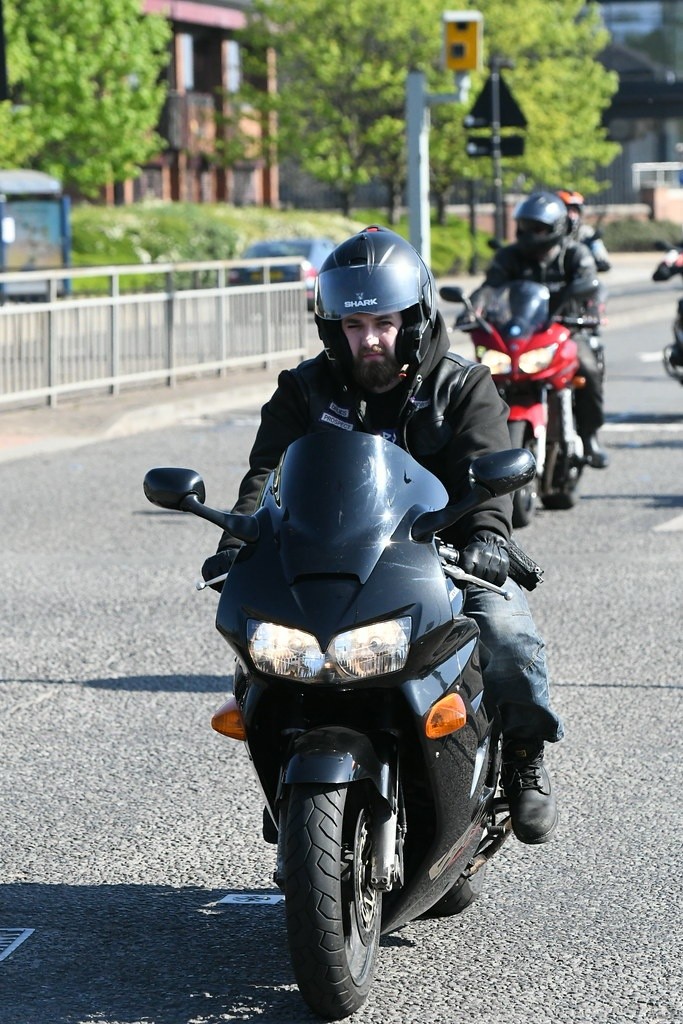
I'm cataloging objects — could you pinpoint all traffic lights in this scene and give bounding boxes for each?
[443,14,480,75]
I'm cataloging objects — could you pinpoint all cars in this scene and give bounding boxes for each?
[230,238,343,312]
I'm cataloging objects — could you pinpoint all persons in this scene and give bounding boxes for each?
[526,188,611,361]
[196,223,565,843]
[457,191,611,472]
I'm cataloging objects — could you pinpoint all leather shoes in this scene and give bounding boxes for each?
[582,435,611,470]
[499,738,559,844]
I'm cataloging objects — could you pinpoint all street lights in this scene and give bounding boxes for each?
[484,44,516,245]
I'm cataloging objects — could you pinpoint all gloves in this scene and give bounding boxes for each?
[202,547,240,594]
[453,530,510,590]
[581,306,602,327]
[456,313,476,334]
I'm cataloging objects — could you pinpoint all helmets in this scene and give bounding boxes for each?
[512,190,570,252]
[555,189,584,230]
[314,224,440,383]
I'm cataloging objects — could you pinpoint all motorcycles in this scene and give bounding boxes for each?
[142,425,530,1020]
[651,235,683,386]
[486,229,608,280]
[439,278,606,530]
